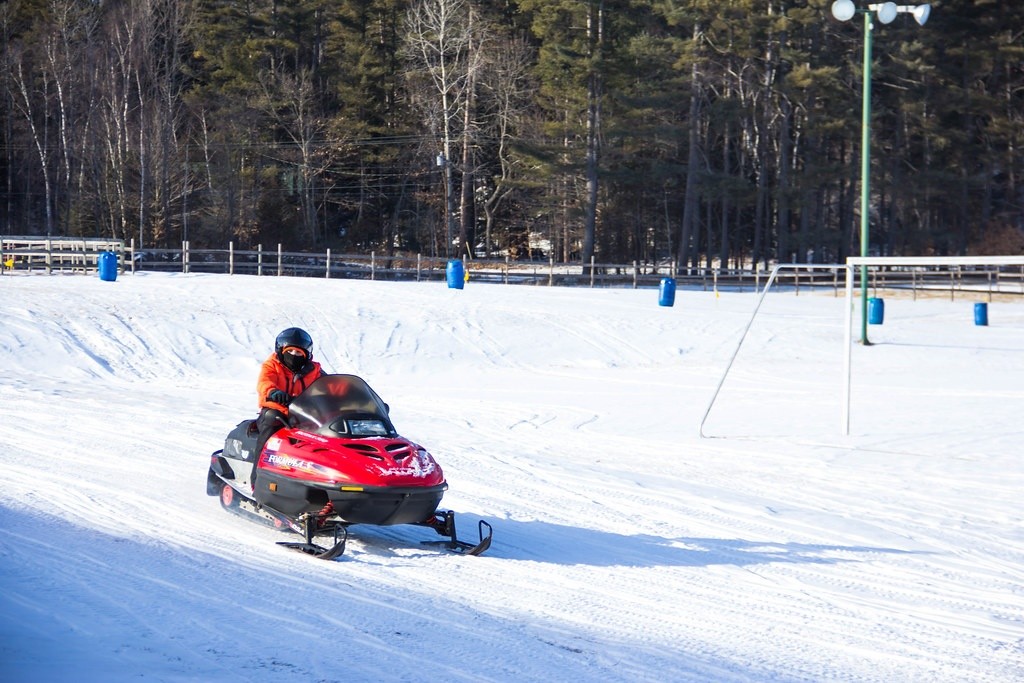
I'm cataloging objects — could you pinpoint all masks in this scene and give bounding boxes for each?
[283,351,305,369]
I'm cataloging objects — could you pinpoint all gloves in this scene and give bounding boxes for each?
[272,390,291,405]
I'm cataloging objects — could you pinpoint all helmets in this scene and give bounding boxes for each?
[275,327,313,361]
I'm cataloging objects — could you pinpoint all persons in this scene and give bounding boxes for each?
[250,327,371,498]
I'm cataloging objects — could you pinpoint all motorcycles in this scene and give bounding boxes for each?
[207,374,495,559]
